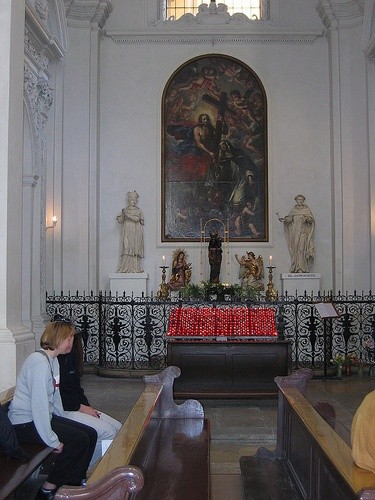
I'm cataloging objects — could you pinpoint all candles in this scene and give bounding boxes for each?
[163,256,166,266]
[269,255,272,267]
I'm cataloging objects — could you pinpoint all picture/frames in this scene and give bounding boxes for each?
[160,54,269,242]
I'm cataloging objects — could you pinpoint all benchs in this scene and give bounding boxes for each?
[56,366,212,500]
[238,369,375,500]
[0,441,56,500]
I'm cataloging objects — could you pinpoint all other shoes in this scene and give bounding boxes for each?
[252,232,264,239]
[39,478,82,499]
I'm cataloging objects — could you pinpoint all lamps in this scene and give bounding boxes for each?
[46,215,57,231]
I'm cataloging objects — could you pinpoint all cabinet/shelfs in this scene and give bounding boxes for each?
[162,334,290,401]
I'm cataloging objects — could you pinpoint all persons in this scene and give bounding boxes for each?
[208,229,223,283]
[115,192,144,273]
[167,251,190,291]
[235,251,263,290]
[283,194,316,273]
[8,321,122,500]
